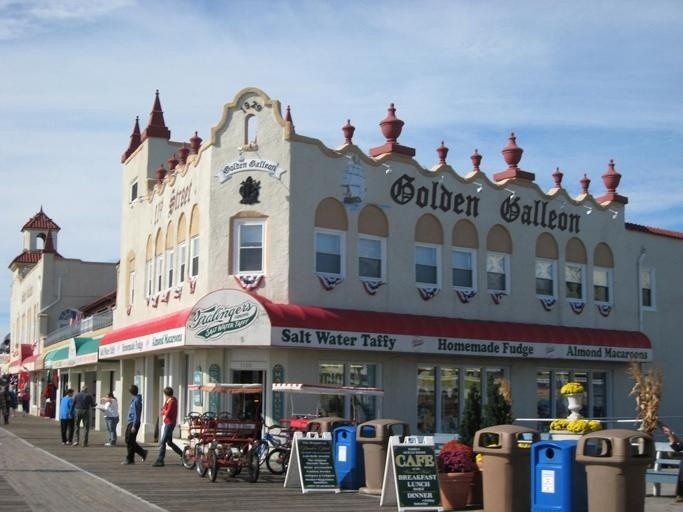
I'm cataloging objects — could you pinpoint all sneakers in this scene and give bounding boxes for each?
[121,449,165,466]
[59,440,118,447]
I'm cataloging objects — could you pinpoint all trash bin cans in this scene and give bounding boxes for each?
[472,425,656,512]
[305,417,410,498]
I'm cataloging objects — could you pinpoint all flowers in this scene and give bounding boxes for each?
[435,438,474,470]
[560,380,585,394]
[550,419,602,432]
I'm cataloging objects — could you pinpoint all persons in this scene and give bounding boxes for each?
[20,387,29,417]
[58,388,77,445]
[0,385,14,425]
[120,384,148,466]
[151,386,182,466]
[7,385,17,419]
[94,393,120,447]
[69,385,96,447]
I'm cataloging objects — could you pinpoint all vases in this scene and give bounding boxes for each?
[436,473,482,510]
[563,393,584,418]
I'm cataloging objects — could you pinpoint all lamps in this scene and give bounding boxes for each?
[556,194,620,221]
[470,180,518,202]
[236,144,251,163]
[379,161,394,176]
